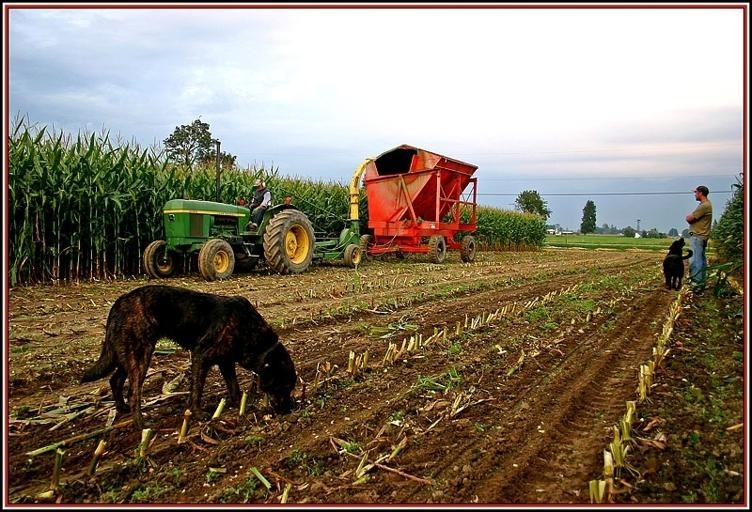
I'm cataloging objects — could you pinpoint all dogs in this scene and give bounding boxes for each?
[663,238,693,291]
[79,284,297,427]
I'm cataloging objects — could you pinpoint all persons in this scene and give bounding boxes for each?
[685,186,712,284]
[247,179,271,230]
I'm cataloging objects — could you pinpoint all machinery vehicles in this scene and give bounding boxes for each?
[141,139,373,279]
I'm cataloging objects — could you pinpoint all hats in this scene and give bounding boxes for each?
[253,178,262,188]
